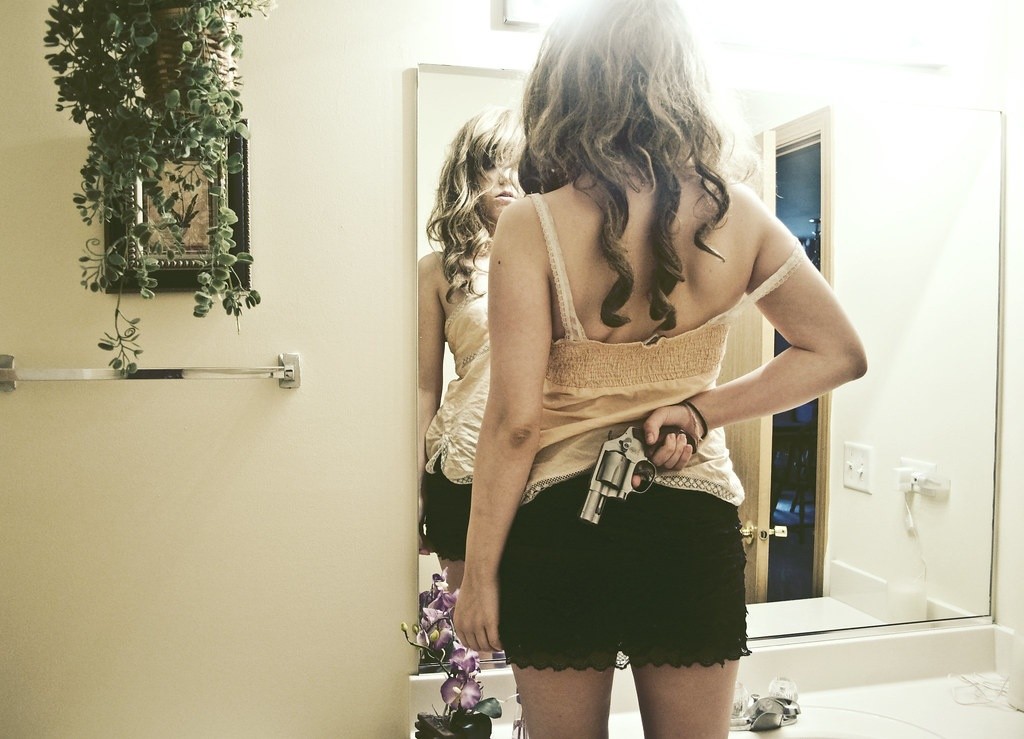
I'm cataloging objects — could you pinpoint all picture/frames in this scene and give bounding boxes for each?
[104,118,252,294]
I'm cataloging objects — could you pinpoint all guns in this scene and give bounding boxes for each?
[577,425,697,529]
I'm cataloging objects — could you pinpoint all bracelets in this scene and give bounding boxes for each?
[683,401,708,446]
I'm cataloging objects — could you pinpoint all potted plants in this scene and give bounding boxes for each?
[41,1,278,378]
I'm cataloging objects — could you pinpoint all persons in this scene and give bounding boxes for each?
[454,0,869,739]
[418,106,528,662]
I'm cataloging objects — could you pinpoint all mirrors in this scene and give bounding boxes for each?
[416,57,1007,678]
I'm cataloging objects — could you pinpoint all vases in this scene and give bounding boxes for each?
[414,714,493,739]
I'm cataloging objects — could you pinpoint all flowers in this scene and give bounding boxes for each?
[420,567,464,663]
[400,606,502,730]
